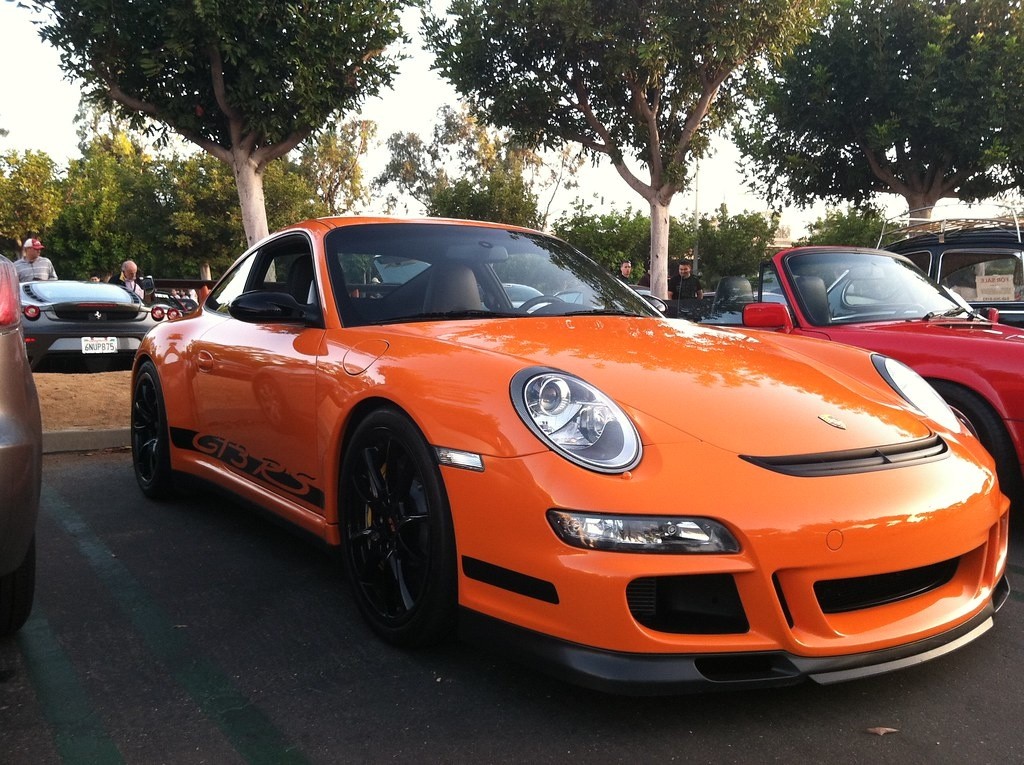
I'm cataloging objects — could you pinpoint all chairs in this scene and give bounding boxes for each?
[287,248,355,328]
[708,277,755,324]
[422,264,486,314]
[794,275,832,326]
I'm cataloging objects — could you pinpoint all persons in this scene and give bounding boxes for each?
[617,260,633,285]
[170,289,189,299]
[668,261,703,301]
[107,261,143,298]
[13,238,58,282]
[90,275,99,282]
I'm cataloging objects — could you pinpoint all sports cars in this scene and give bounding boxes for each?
[655,245,1024,522]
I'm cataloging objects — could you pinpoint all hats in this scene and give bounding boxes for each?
[22,238,44,250]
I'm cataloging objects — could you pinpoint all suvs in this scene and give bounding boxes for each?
[875,204,1024,328]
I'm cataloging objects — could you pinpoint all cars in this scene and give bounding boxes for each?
[0,255,42,631]
[154,290,198,317]
[19,280,183,374]
[129,216,1011,686]
